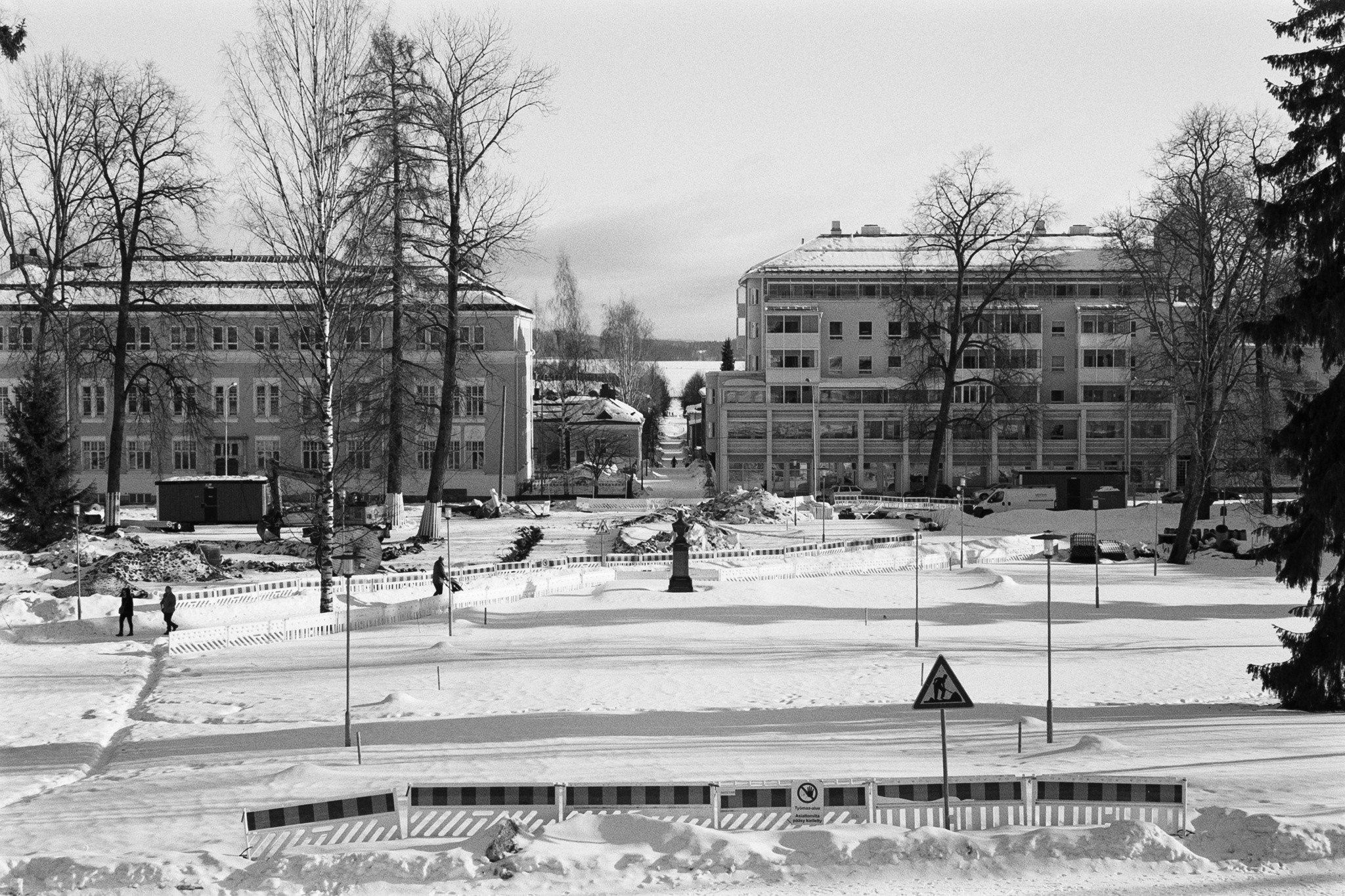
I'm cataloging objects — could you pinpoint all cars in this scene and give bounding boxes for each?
[280,504,316,526]
[972,482,1013,501]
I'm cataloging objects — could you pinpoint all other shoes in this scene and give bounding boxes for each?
[172,625,179,631]
[127,632,133,636]
[164,630,171,635]
[116,631,124,637]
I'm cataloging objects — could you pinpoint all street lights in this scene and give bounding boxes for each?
[905,512,932,646]
[956,474,971,568]
[439,503,461,636]
[1088,495,1104,608]
[1030,529,1069,744]
[806,378,817,501]
[332,548,366,747]
[225,382,238,475]
[1151,477,1165,576]
[74,501,82,620]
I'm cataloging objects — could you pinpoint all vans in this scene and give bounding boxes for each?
[973,483,1058,518]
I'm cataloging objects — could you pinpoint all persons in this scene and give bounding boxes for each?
[267,504,273,514]
[671,457,678,467]
[116,588,134,636]
[433,556,448,596]
[672,510,689,536]
[160,586,178,635]
[761,480,767,490]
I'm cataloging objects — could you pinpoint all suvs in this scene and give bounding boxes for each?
[830,484,863,500]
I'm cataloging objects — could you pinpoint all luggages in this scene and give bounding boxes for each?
[444,578,463,592]
